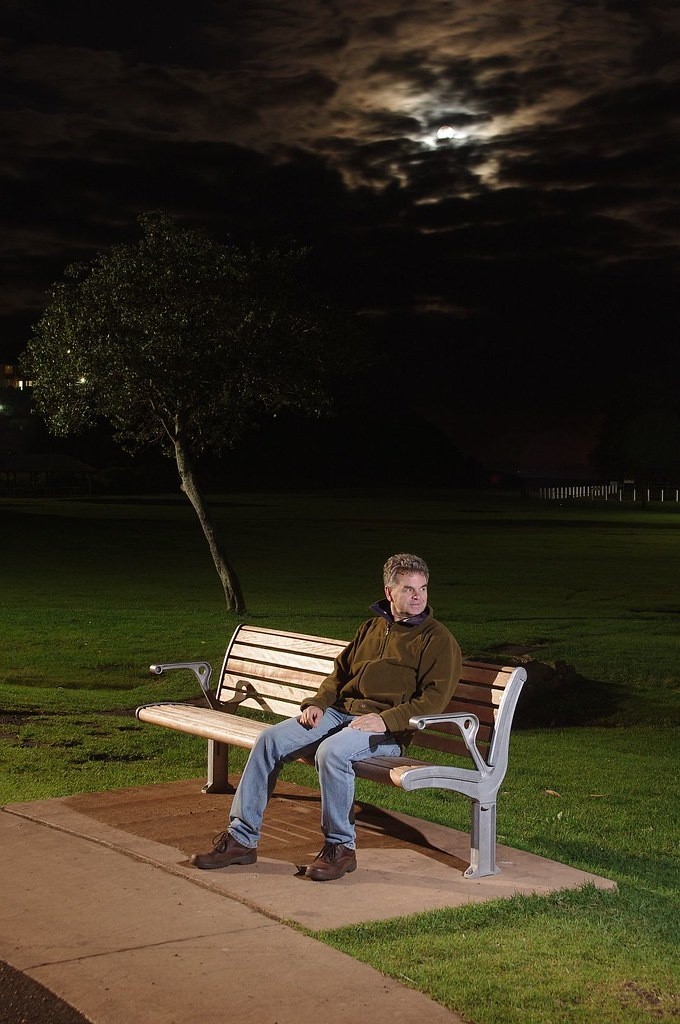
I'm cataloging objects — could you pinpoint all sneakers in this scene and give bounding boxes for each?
[306,842,358,881]
[189,830,256,869]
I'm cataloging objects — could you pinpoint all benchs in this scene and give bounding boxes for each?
[134,623,528,878]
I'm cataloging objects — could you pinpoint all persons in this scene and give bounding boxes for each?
[190,554,463,880]
[490,471,505,497]
